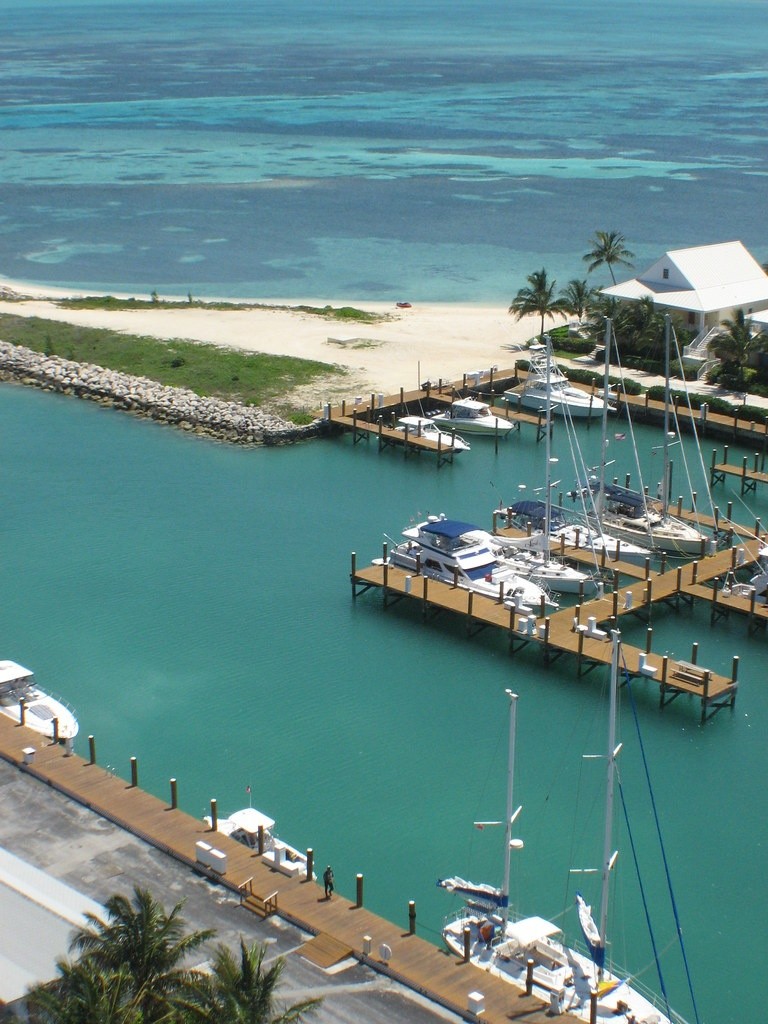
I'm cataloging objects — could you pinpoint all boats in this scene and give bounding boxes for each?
[504,334,617,417]
[430,397,514,437]
[397,302,411,308]
[389,513,562,611]
[0,658,81,742]
[722,546,768,606]
[394,414,471,453]
[202,809,318,886]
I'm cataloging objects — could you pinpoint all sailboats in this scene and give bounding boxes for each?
[439,628,701,1024]
[487,313,721,595]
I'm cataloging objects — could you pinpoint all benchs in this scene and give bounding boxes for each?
[670,668,703,687]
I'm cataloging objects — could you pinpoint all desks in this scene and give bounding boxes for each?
[674,660,710,678]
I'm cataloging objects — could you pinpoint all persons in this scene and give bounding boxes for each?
[323,866,334,898]
